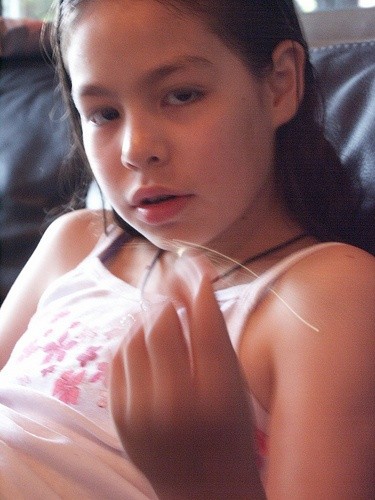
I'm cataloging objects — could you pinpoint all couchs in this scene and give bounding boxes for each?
[0,40,375,306]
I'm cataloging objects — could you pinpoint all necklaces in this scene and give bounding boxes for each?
[139,231,315,317]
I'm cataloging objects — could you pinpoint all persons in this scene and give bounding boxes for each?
[0,0,374,499]
